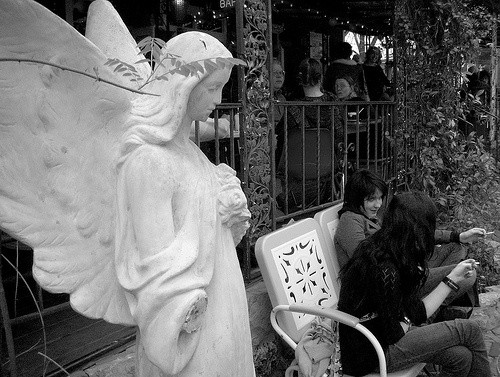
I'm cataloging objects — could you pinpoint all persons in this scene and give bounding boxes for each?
[338,190,491,377]
[0,0,255,377]
[264,42,489,211]
[334,170,486,307]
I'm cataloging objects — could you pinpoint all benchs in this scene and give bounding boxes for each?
[256,202,427,377]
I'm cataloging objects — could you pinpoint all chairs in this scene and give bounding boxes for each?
[276,128,335,209]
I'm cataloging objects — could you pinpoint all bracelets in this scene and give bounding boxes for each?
[442,277,460,292]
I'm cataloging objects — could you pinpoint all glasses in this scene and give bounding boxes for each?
[273,70,286,76]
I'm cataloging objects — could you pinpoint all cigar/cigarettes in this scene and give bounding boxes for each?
[475,261,481,265]
[485,232,495,234]
